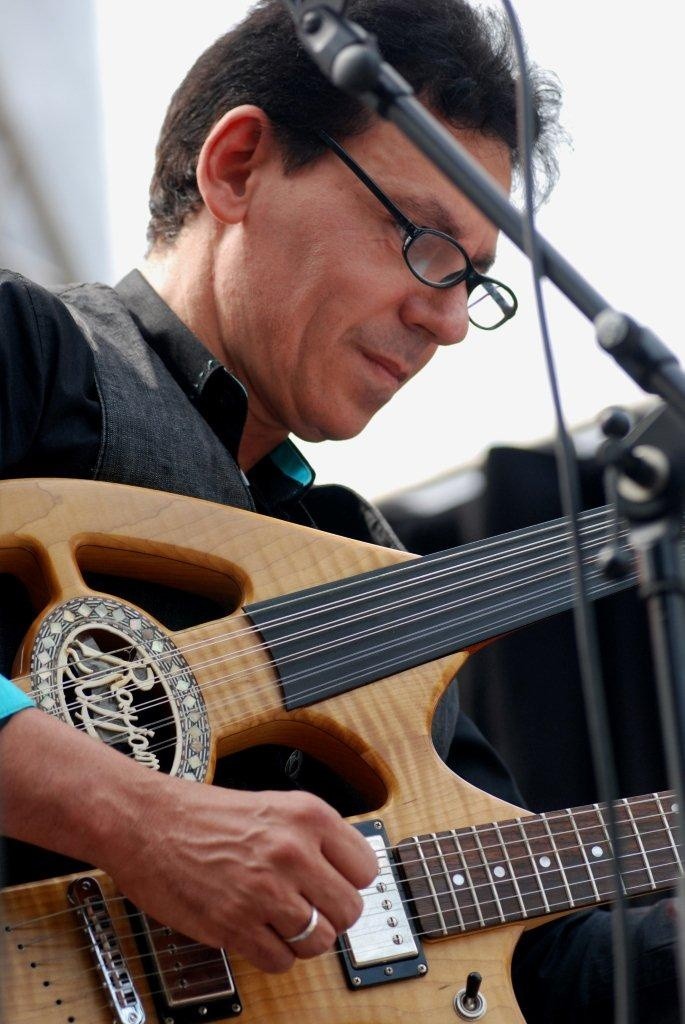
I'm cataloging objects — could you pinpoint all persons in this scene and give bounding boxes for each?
[0,2,684,1022]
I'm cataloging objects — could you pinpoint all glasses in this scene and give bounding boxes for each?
[259,108,518,333]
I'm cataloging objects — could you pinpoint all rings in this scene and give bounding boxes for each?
[284,906,319,943]
